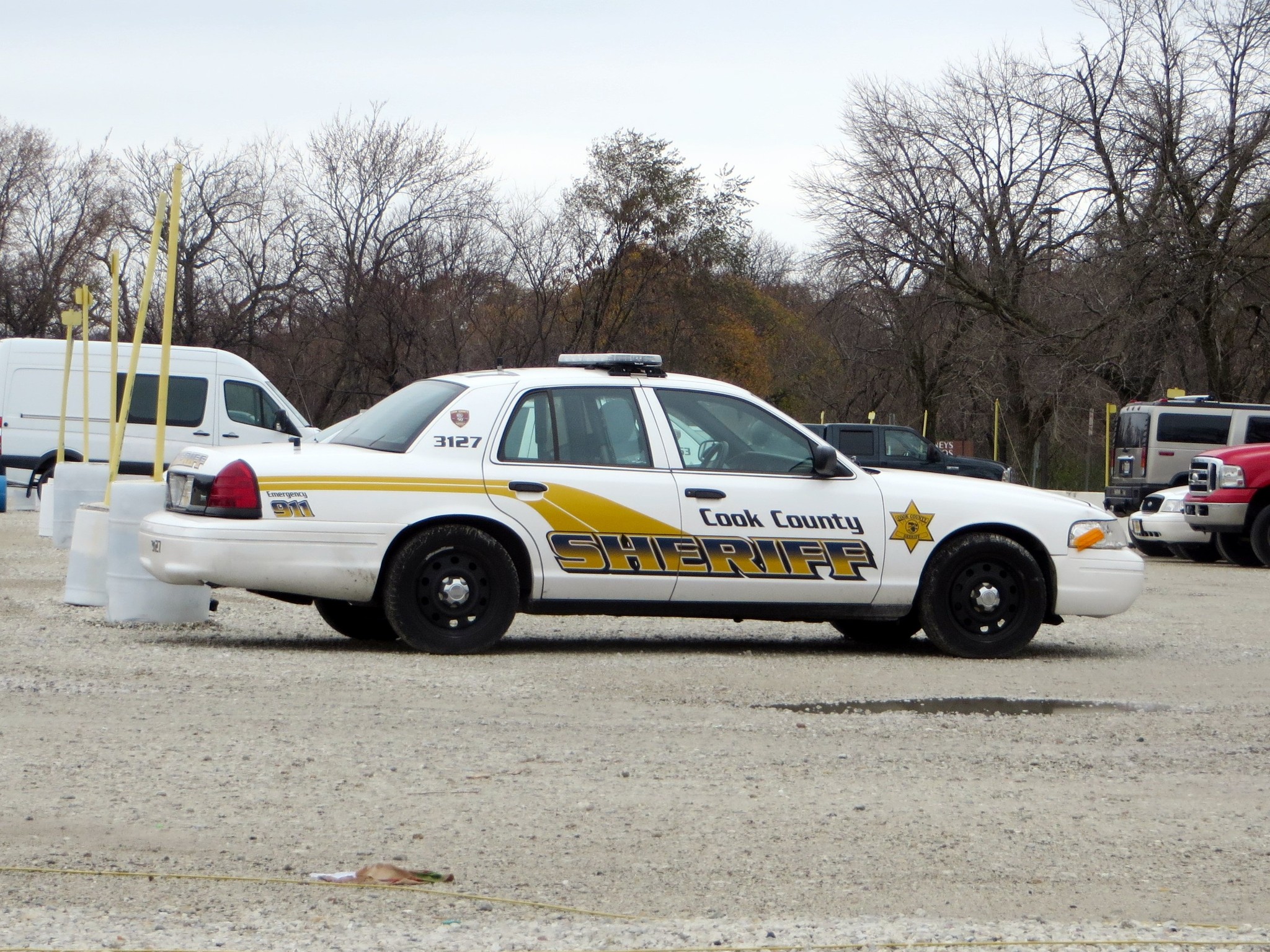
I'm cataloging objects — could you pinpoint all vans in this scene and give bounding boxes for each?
[2,338,321,514]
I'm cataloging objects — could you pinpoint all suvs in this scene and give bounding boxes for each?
[1179,440,1270,568]
[1101,393,1269,519]
[760,421,1019,489]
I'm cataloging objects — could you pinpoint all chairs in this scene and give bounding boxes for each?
[591,399,656,465]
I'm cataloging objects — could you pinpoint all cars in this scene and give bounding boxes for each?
[136,350,1148,663]
[1129,479,1223,565]
